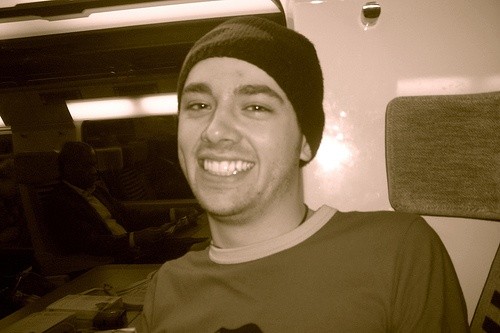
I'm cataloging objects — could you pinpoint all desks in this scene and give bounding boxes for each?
[0,263,163,333]
[156,210,211,255]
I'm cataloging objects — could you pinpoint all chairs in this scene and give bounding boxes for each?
[386,94,500,333]
[15,141,211,277]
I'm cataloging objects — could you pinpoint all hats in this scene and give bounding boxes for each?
[177,15,325,168]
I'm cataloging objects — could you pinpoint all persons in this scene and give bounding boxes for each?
[47,139,184,265]
[140,14,471,333]
